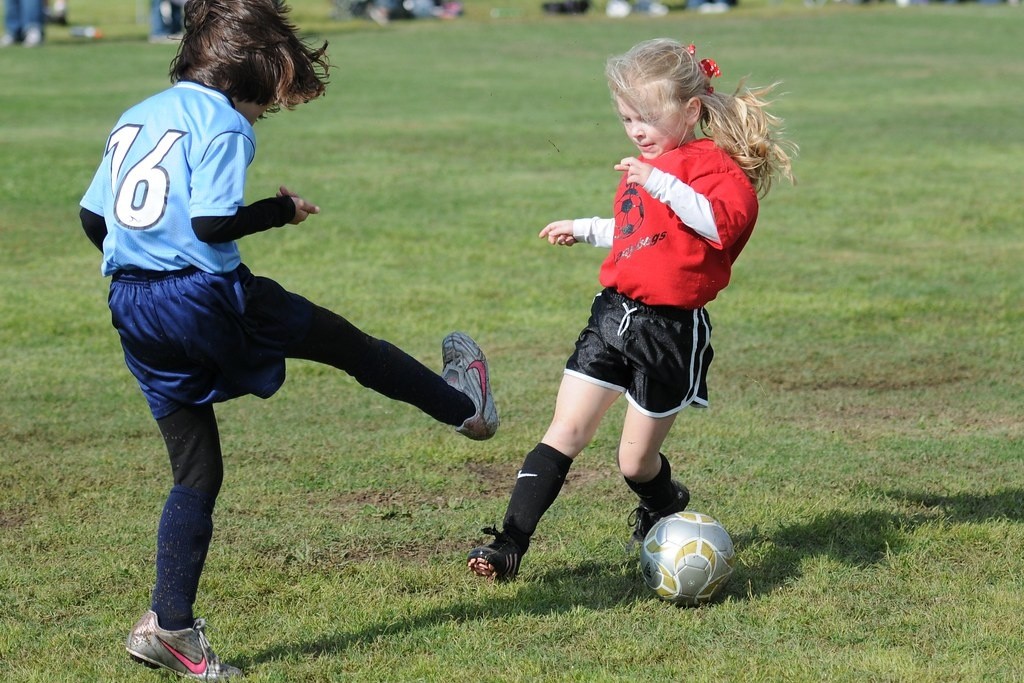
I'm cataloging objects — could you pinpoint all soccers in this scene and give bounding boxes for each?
[638,508,738,607]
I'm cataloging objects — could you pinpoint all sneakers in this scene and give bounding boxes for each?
[0,0,734,49]
[125,609,244,683]
[466,523,523,583]
[439,330,499,440]
[623,479,690,555]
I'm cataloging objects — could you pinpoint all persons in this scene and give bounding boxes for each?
[467,38,792,582]
[150,0,189,44]
[333,0,465,24]
[0,0,68,47]
[76,0,497,676]
